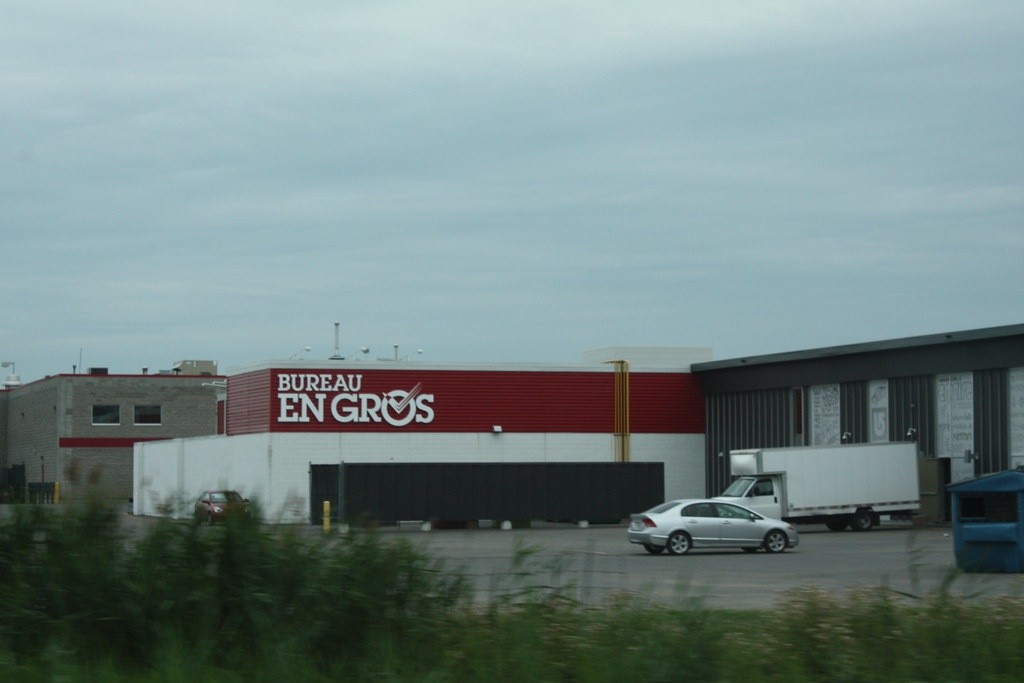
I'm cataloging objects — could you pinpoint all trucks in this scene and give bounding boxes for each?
[716,441,923,533]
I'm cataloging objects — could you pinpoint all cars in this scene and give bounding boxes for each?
[627,497,800,557]
[194,490,251,527]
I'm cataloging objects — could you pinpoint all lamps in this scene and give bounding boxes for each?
[492,425,502,432]
